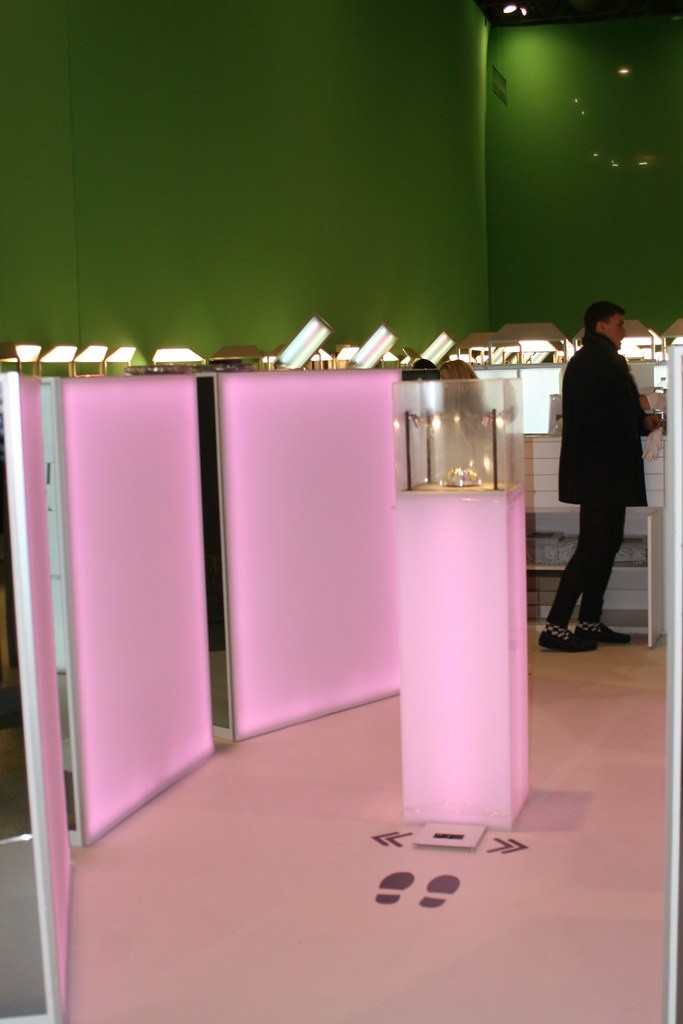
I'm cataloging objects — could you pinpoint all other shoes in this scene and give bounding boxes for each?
[537,624,597,651]
[574,621,631,644]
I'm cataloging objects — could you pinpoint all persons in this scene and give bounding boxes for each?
[413,357,486,434]
[537,300,663,652]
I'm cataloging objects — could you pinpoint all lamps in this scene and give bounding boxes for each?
[1,311,683,371]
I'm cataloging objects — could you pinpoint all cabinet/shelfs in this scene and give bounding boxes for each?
[520,434,664,648]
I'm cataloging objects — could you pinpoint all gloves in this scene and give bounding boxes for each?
[642,425,665,461]
